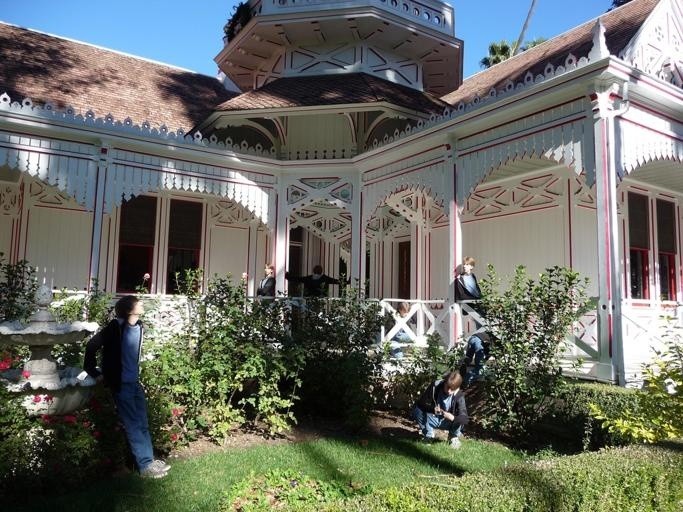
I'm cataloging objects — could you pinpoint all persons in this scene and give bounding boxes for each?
[257,264,275,296]
[460,331,494,375]
[455,256,482,315]
[285,265,351,298]
[83,295,171,478]
[414,372,470,450]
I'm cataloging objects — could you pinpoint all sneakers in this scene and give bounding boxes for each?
[138,461,171,479]
[449,438,459,449]
[419,433,435,441]
